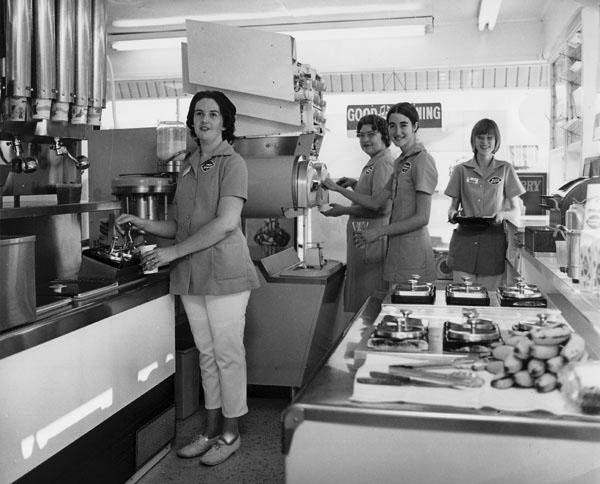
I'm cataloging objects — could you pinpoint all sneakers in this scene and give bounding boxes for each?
[201,432,242,465]
[176,435,222,458]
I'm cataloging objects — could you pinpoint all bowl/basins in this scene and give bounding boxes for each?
[453,216,496,232]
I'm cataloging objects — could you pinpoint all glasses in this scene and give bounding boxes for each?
[359,132,378,138]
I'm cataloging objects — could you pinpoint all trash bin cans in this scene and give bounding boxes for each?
[176,344,200,421]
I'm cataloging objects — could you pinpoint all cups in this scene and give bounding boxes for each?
[555,239,569,266]
[351,221,370,249]
[136,243,160,275]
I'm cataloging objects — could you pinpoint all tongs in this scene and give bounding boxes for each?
[388,355,486,390]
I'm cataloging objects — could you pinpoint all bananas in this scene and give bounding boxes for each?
[471,323,586,393]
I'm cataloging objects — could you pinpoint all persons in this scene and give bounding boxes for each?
[109,88,261,466]
[319,99,438,288]
[441,118,528,291]
[319,112,396,313]
